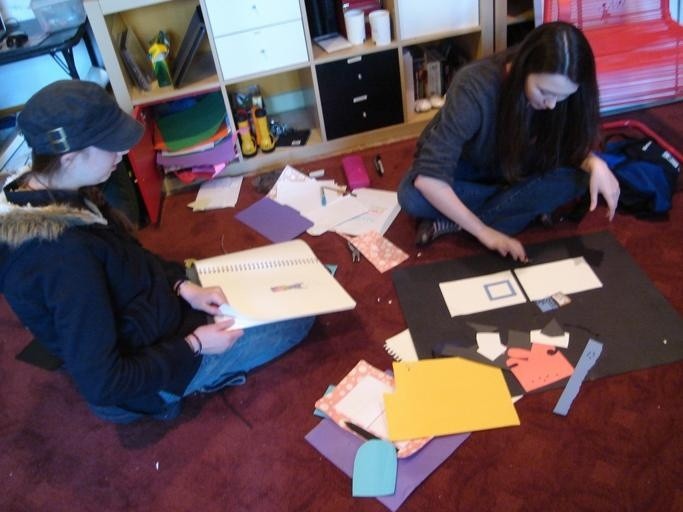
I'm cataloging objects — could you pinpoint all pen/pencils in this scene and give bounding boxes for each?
[325,186,346,194]
[345,422,399,452]
[377,154,385,175]
[321,186,326,206]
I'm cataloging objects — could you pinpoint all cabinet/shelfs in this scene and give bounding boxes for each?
[82,0,509,227]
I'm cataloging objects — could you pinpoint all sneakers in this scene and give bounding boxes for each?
[416,217,465,249]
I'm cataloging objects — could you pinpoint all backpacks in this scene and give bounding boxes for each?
[592,133,680,221]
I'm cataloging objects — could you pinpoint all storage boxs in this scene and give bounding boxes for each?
[28,1,86,33]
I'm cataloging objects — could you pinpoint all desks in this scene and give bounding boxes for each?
[0,18,112,95]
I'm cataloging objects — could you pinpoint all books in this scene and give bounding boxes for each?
[180,235,357,339]
[172,5,206,89]
[400,40,468,113]
[117,26,153,93]
[313,32,352,55]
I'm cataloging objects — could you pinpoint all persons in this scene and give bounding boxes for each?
[395,20,621,263]
[0,81,317,424]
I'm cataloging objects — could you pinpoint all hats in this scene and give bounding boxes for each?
[18,81,144,154]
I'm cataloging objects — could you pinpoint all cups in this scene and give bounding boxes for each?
[344,9,366,45]
[369,10,392,47]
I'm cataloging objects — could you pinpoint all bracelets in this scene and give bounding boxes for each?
[188,329,202,360]
[175,279,185,295]
